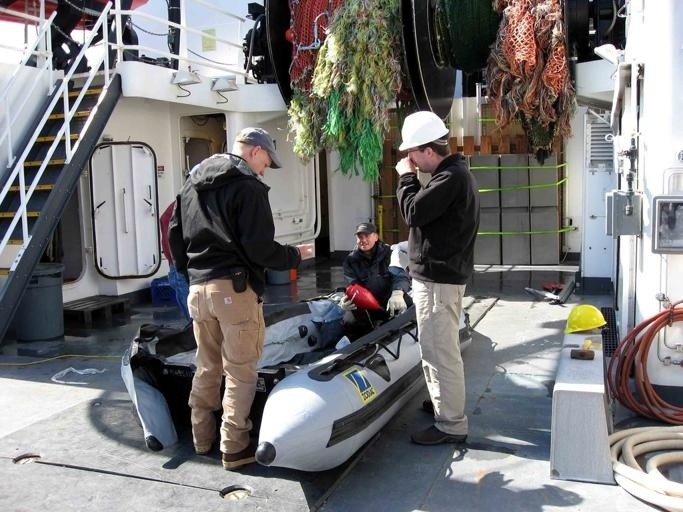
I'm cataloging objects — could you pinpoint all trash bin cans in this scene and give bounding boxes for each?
[12,264,67,343]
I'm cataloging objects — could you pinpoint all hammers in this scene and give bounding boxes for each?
[570,339,595,360]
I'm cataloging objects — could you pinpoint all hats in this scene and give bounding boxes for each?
[236,127,282,169]
[353,222,376,236]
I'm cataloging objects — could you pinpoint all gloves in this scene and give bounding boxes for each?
[339,294,357,311]
[386,289,408,316]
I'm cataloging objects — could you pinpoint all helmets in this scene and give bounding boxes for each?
[397,110,450,152]
[562,304,608,334]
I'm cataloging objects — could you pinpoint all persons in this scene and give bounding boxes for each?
[156,200,202,324]
[392,110,482,446]
[139,286,387,370]
[340,222,413,332]
[161,126,315,470]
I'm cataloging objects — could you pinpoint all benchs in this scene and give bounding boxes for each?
[63,295,132,337]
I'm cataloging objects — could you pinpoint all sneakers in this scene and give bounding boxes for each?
[221,440,258,470]
[423,399,436,416]
[195,438,221,457]
[410,424,467,445]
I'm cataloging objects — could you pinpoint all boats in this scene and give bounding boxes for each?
[120,287,473,472]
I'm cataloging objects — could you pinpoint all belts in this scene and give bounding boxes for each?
[214,274,232,280]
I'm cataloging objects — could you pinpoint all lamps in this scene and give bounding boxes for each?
[212,74,238,104]
[171,66,201,98]
